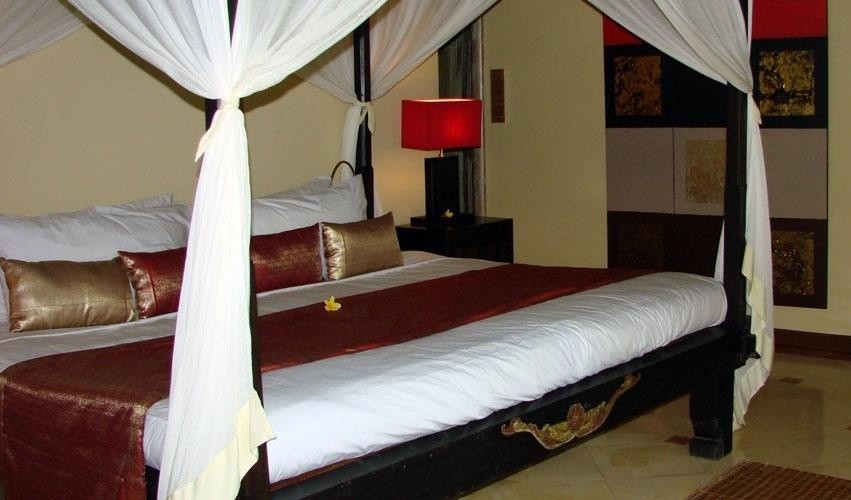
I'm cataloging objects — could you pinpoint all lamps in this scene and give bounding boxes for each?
[398,97,483,216]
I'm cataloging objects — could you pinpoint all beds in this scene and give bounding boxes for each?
[0,0,777,500]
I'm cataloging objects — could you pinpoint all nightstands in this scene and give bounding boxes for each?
[395,215,514,264]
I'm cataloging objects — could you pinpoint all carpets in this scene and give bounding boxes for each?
[691,463,851,499]
